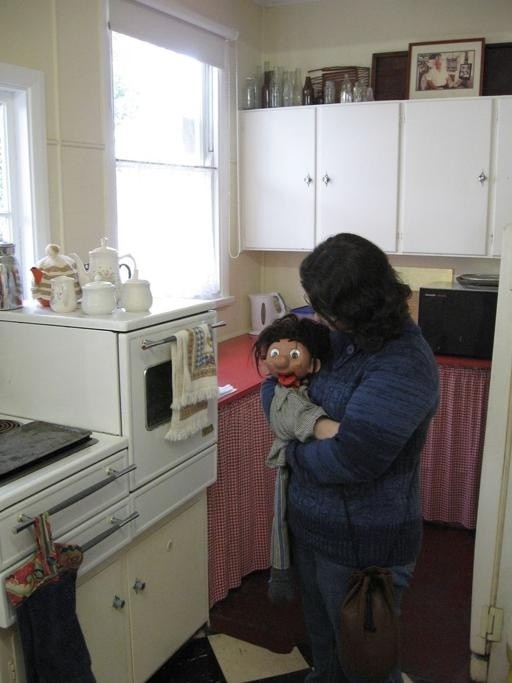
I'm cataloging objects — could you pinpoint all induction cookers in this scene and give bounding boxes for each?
[1,412,129,511]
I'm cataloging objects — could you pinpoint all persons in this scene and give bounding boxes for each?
[260,232,442,683]
[251,313,332,387]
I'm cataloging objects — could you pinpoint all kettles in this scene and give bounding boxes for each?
[247,291,288,336]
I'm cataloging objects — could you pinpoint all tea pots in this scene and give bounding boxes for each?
[29,241,83,308]
[68,236,136,306]
[49,274,82,315]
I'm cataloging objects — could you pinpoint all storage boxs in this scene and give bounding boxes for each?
[289,303,316,320]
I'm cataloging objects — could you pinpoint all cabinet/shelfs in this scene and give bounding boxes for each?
[1,487,209,682]
[229,98,397,256]
[397,93,512,259]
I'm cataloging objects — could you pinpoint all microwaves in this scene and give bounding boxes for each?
[418,279,498,361]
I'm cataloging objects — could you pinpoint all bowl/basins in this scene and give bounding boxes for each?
[456,272,499,290]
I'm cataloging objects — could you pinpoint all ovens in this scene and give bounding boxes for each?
[1,297,228,492]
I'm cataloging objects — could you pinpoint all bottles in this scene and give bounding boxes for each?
[239,60,374,110]
[0,240,25,310]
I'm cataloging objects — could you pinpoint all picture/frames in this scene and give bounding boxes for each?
[407,35,486,96]
[371,41,511,102]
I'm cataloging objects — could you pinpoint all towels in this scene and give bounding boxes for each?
[165,325,222,440]
